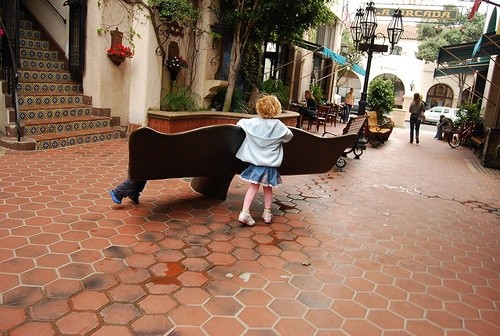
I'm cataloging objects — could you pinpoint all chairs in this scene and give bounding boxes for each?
[289,101,339,132]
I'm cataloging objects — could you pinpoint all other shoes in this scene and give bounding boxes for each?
[110,191,121,204]
[128,195,139,204]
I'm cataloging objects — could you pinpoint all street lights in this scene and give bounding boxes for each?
[350,0,404,116]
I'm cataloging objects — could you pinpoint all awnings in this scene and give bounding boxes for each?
[265,36,366,85]
[433,32,500,105]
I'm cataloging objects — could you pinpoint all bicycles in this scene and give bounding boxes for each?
[447,121,478,149]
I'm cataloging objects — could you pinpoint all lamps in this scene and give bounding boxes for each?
[410,80,414,91]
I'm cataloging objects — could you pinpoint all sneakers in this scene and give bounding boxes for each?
[237,211,256,226]
[262,212,272,223]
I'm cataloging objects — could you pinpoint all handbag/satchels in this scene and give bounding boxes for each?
[421,111,425,123]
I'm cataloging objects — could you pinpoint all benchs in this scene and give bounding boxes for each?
[323,115,368,169]
[470,130,489,158]
[365,110,391,148]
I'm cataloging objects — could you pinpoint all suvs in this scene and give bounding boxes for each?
[421,106,461,125]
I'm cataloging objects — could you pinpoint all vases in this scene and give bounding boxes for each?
[110,55,125,64]
[168,67,182,73]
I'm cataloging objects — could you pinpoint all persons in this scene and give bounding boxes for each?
[409,93,426,144]
[235,95,293,226]
[296,91,317,128]
[344,88,356,122]
[435,115,452,138]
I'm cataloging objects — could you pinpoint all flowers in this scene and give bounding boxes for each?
[164,56,187,67]
[106,44,131,57]
